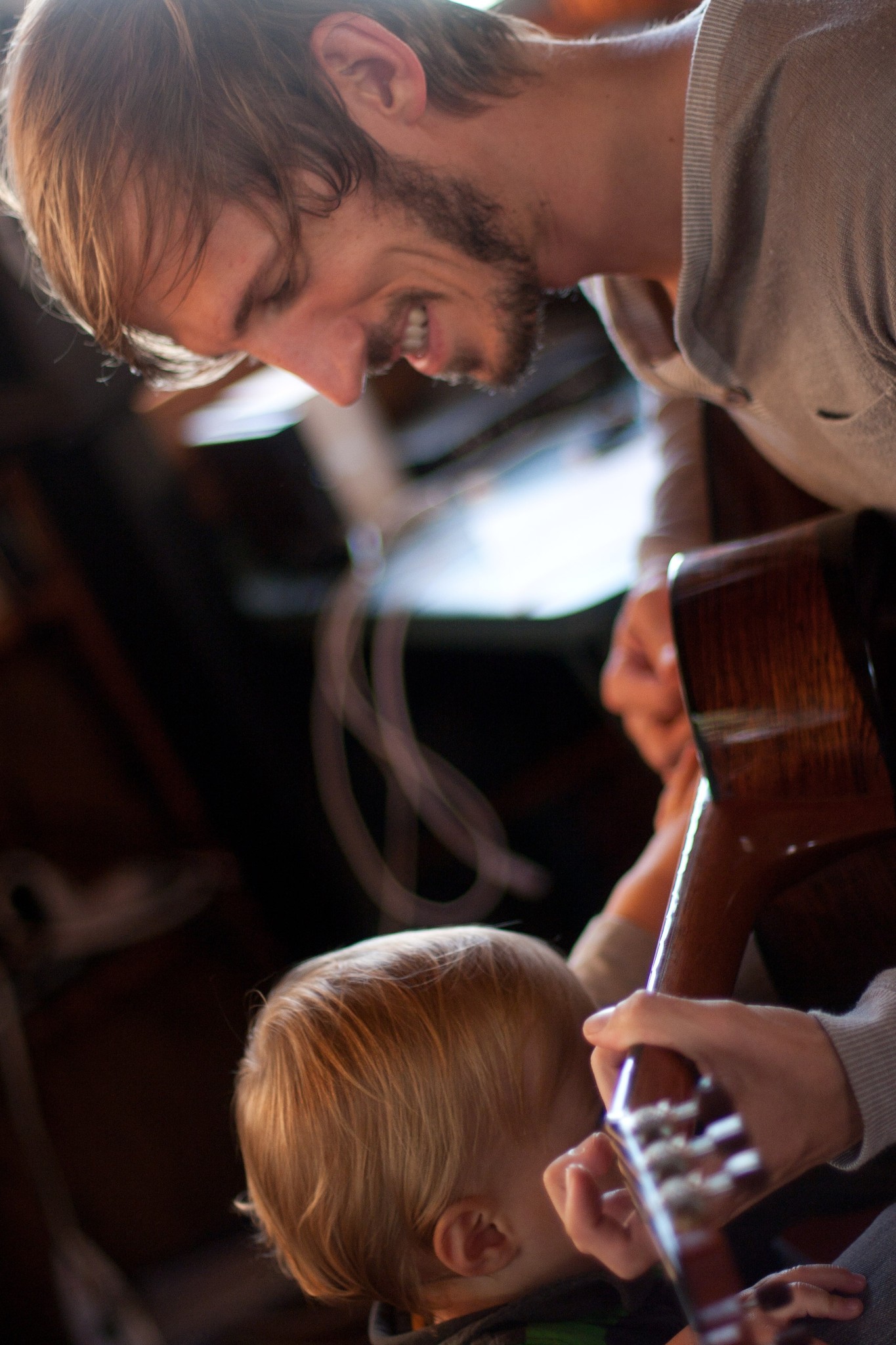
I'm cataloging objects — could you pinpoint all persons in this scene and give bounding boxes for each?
[0,0,896,1345]
[234,924,870,1345]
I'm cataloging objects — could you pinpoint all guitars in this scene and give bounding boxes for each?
[603,400,896,1345]
